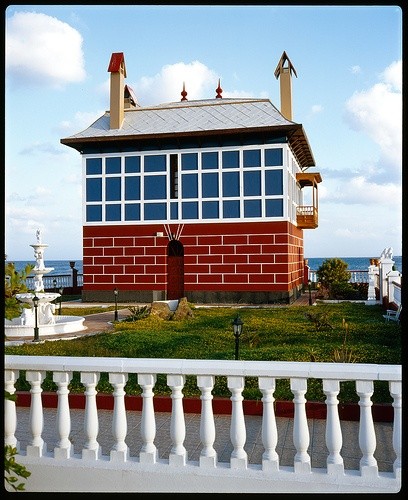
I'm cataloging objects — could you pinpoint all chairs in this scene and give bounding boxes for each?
[382,304,402,324]
